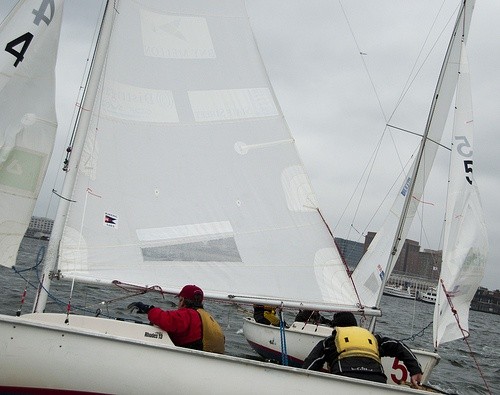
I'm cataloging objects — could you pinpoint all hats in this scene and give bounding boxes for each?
[329,313,357,327]
[174,285,203,303]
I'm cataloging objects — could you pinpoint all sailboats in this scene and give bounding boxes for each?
[0,0,444,395]
[235,0,489,391]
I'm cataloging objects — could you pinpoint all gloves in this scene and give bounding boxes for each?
[128,302,155,314]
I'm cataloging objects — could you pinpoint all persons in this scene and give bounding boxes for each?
[301,312,423,390]
[128,285,225,354]
[253,304,333,328]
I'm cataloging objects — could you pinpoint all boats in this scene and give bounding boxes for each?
[383,283,437,304]
